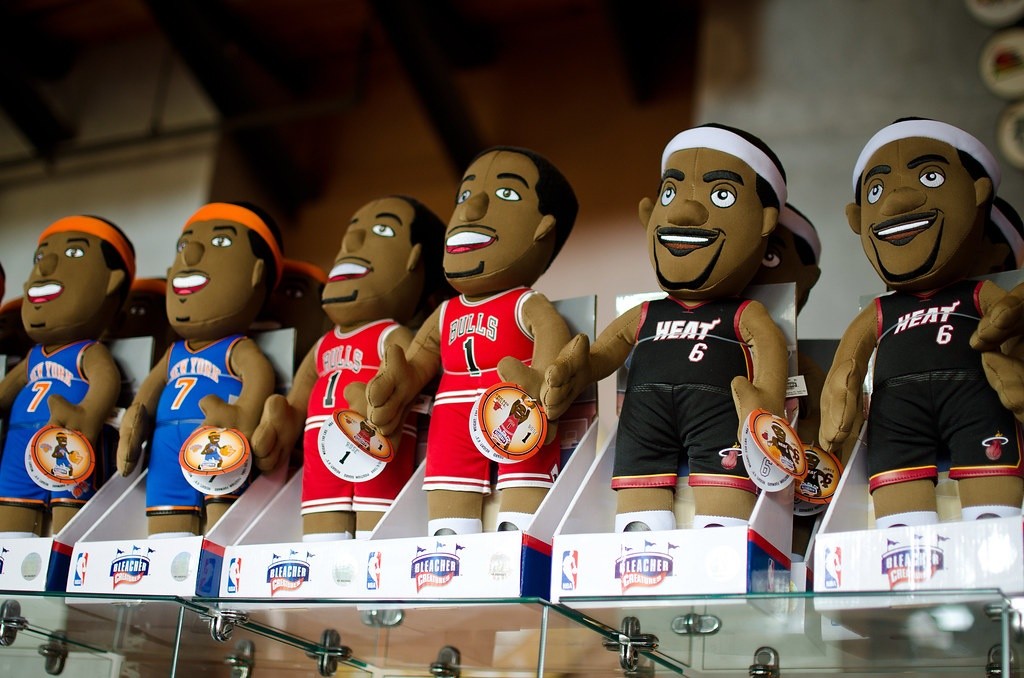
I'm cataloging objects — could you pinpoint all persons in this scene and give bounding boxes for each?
[820,115,1024,528]
[0,215,135,538]
[252,196,445,541]
[115,201,282,541]
[366,146,580,535]
[540,123,789,534]
